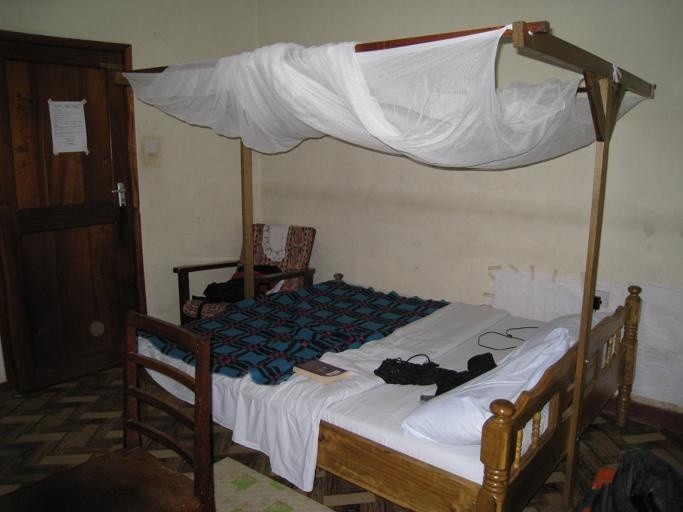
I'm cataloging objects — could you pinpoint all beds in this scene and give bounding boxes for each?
[114,20,656,512]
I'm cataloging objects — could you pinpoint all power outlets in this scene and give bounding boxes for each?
[595,290,608,308]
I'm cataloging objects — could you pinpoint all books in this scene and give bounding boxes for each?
[291,359,349,385]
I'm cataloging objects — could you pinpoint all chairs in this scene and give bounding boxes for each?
[0,311,216,512]
[173,224,315,325]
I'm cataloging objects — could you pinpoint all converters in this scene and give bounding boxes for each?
[592,296,601,310]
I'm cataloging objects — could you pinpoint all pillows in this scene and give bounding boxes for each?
[403,327,569,447]
[522,313,607,347]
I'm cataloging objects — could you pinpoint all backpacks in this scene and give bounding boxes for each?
[569,441,683,512]
[204,262,283,303]
